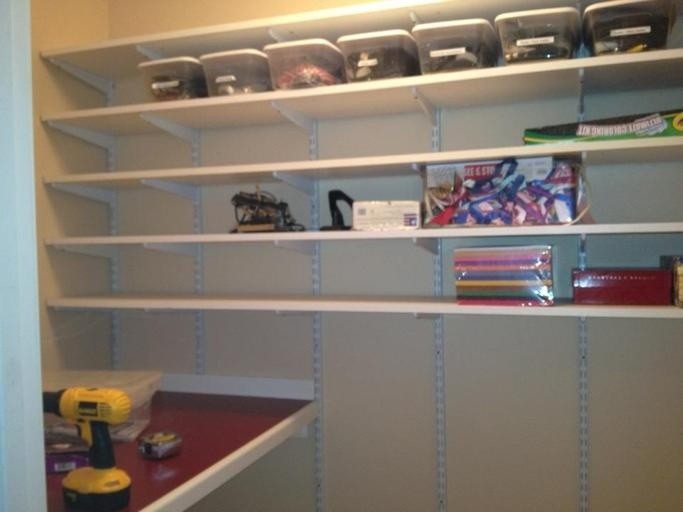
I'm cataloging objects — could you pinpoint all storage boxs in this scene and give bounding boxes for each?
[41,368,163,442]
[199,48,267,99]
[136,57,205,103]
[582,0,676,56]
[264,38,342,91]
[495,7,581,64]
[337,29,417,82]
[571,267,674,305]
[411,18,499,75]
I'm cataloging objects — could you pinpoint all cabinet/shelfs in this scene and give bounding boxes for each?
[28,0,683,319]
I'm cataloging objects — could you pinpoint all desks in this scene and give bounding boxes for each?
[46,391,319,512]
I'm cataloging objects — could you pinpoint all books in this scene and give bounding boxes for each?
[452,244,553,307]
